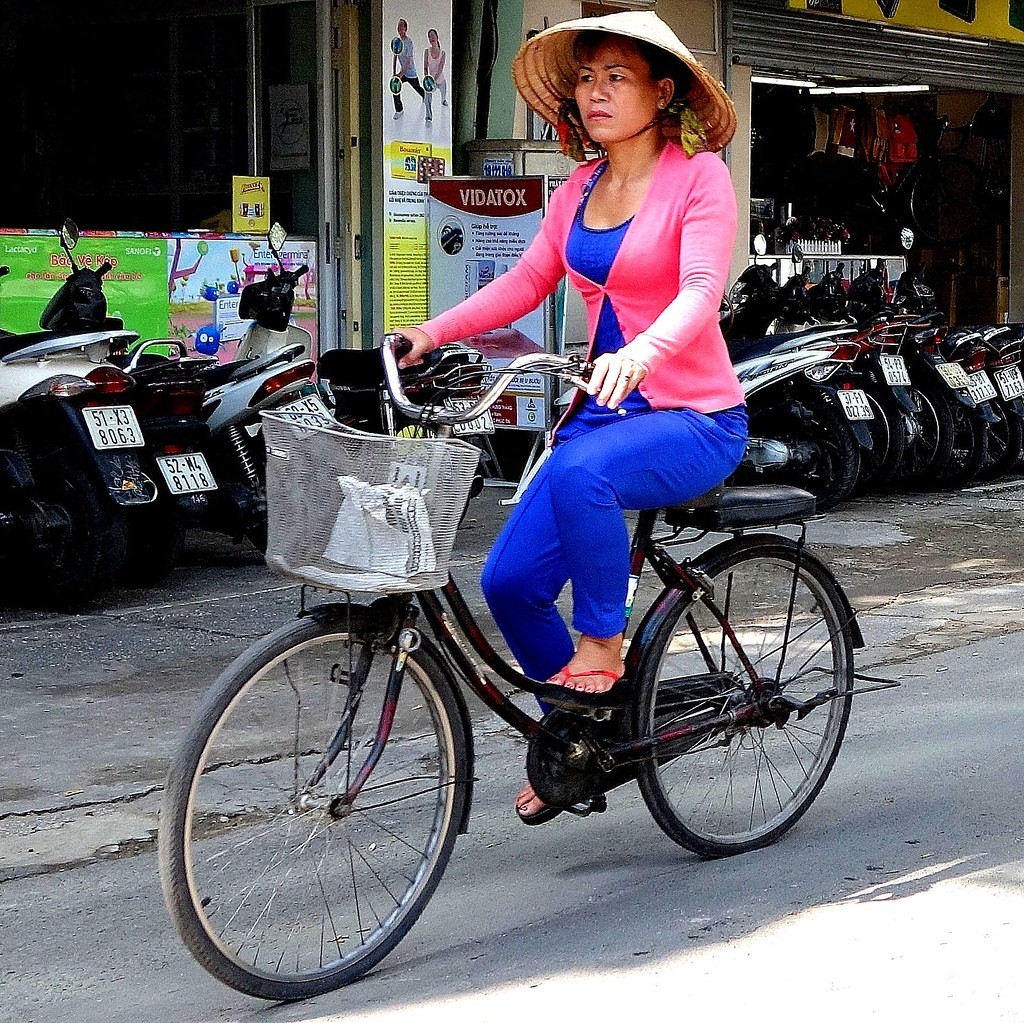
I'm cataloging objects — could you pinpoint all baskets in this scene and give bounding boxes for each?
[258,409,483,596]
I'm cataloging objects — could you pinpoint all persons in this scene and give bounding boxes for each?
[419,29,448,128]
[390,11,749,825]
[389,19,424,120]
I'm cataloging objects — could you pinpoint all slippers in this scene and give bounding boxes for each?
[514,784,566,826]
[536,666,629,709]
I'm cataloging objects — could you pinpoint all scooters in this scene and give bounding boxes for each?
[0,216,1024,611]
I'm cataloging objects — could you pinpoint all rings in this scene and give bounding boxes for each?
[620,375,631,381]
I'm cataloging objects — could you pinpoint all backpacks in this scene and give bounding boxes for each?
[886,109,918,164]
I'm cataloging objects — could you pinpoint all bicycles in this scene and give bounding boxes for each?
[156,328,901,1003]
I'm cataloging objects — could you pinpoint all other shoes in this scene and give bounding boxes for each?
[443,101,448,107]
[393,110,404,119]
[425,117,432,126]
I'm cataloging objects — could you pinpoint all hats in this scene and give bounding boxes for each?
[513,11,738,154]
